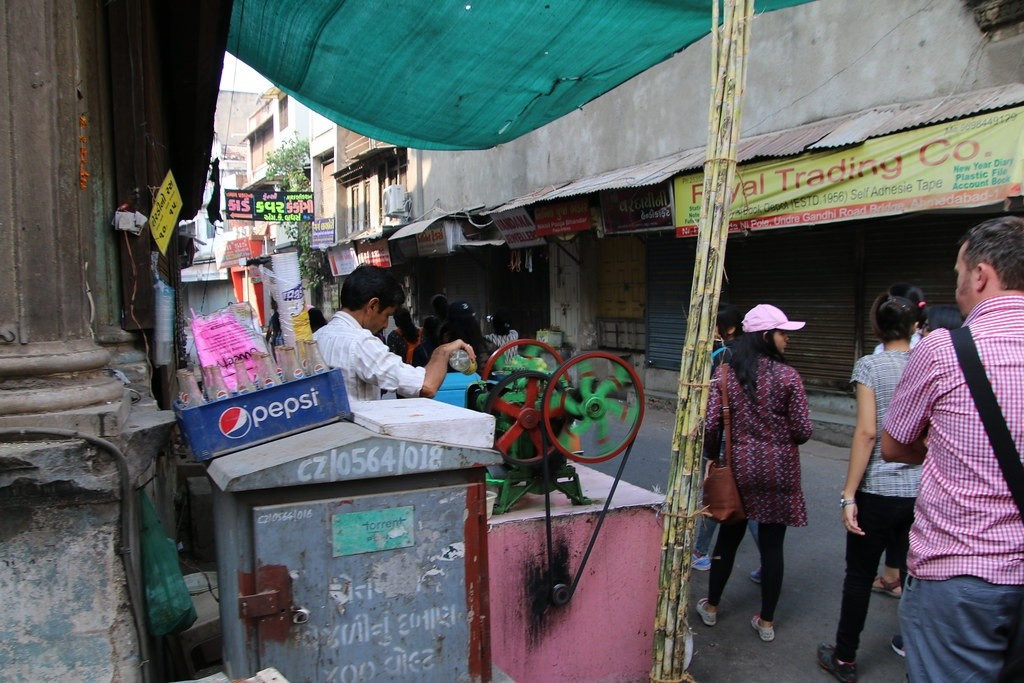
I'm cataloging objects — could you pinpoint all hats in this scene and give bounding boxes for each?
[741,304,806,333]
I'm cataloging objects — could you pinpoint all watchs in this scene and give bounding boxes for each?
[840,499,854,510]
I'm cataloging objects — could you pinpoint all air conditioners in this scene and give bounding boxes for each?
[381,184,410,219]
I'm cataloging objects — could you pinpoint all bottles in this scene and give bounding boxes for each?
[449,349,477,376]
[176,339,330,409]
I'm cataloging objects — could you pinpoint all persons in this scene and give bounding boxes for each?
[422,294,482,357]
[483,314,518,360]
[386,308,425,399]
[312,265,476,402]
[696,304,813,641]
[816,293,922,683]
[867,283,926,598]
[881,217,1024,683]
[266,295,284,345]
[689,310,759,580]
[307,304,327,334]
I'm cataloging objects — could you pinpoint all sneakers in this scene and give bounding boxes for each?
[816,642,857,683]
[691,549,711,570]
[751,614,775,641]
[696,598,716,625]
[750,564,762,583]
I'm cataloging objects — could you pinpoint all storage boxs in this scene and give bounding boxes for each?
[174,364,353,461]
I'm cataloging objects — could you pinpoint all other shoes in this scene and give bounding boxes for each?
[891,635,906,657]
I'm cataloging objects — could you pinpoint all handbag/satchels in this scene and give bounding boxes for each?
[995,592,1024,683]
[133,491,198,637]
[700,460,745,523]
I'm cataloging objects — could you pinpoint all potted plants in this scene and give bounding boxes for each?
[536,325,563,349]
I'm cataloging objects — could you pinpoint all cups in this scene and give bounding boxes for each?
[153,288,175,366]
[271,251,313,356]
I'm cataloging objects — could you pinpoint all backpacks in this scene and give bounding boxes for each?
[395,327,430,367]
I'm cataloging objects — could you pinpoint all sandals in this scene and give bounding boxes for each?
[871,576,902,598]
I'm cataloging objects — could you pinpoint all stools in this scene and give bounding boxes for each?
[165,586,221,681]
